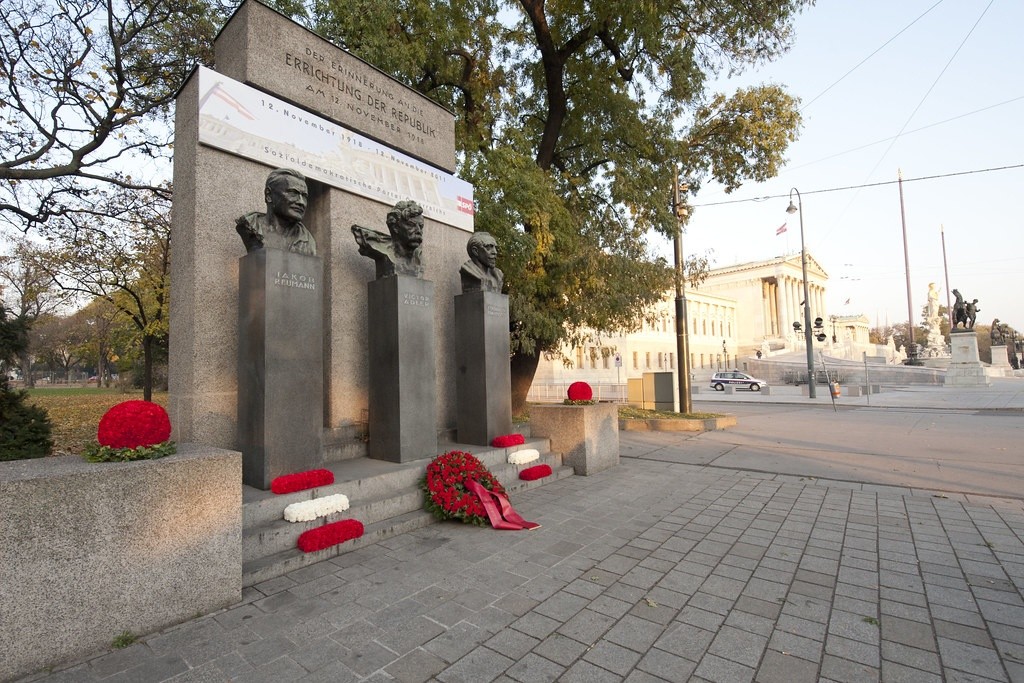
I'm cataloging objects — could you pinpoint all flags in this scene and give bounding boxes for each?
[845,299,849,305]
[776,223,786,236]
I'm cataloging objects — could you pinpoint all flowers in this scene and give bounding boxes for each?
[417,449,510,527]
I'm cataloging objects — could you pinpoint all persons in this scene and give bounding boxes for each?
[459,231,503,293]
[964,299,980,329]
[386,200,424,259]
[235,169,316,256]
[998,324,1009,342]
[928,283,939,316]
[756,351,762,359]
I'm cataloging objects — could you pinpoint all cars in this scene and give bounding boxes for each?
[90,376,97,380]
[110,374,118,379]
[711,371,767,392]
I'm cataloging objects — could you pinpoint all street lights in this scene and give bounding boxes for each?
[785,187,815,398]
